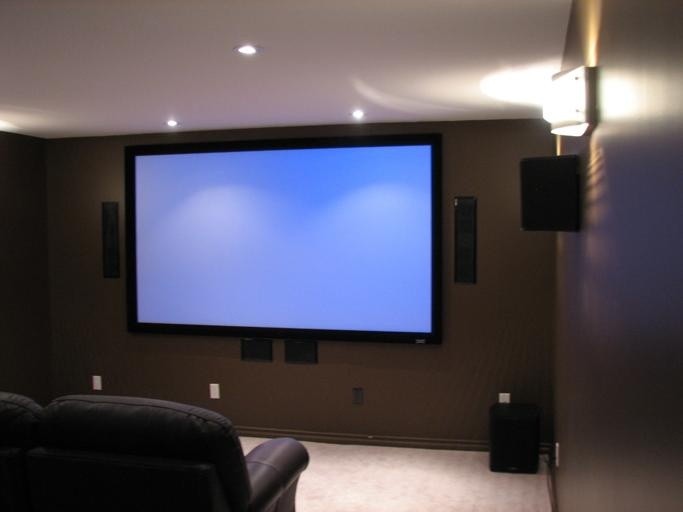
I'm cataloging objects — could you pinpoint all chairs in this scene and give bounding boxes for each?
[0,390,42,511]
[35,390,309,511]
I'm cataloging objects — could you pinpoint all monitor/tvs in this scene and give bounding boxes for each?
[124,132,442,345]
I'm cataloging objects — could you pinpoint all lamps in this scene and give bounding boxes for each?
[549,63,598,139]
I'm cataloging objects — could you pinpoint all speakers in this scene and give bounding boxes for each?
[521,155,581,232]
[454,197,477,283]
[241,339,273,361]
[286,341,317,362]
[103,202,121,278]
